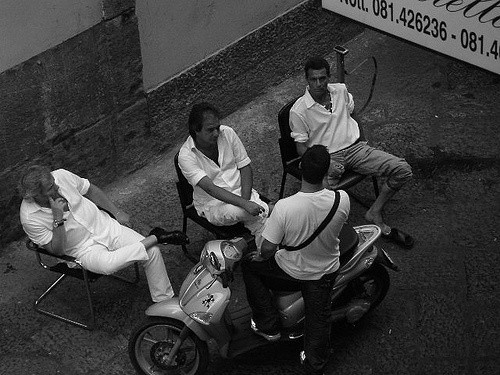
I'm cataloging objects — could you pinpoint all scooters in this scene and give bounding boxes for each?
[128,223,401,375]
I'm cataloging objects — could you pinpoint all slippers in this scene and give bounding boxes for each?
[381,228,414,249]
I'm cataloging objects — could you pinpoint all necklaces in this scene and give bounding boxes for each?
[309,89,332,111]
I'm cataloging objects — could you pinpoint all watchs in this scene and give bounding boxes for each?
[52,219,67,228]
[259,251,268,260]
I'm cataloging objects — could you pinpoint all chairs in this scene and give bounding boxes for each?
[276,94,383,202]
[27,207,140,331]
[173,153,255,264]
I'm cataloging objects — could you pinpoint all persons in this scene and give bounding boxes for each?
[242,144,351,375]
[20,165,191,336]
[179,101,281,255]
[289,55,414,250]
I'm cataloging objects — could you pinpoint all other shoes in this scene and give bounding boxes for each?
[168,321,193,339]
[149,227,189,245]
[251,317,281,341]
[300,350,306,364]
